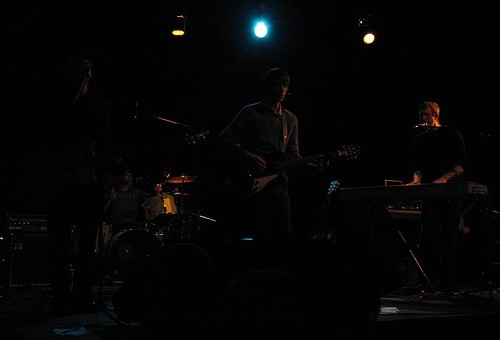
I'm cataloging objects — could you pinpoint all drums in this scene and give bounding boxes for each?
[105,228,150,281]
[142,193,179,224]
[147,226,169,241]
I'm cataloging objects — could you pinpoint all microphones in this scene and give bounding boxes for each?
[414,122,426,128]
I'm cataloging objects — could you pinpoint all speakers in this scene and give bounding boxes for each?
[0,234,52,287]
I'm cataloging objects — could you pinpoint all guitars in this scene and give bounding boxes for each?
[234,144,360,196]
[320,180,340,222]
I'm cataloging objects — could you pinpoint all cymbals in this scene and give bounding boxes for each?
[164,175,195,184]
[164,191,190,196]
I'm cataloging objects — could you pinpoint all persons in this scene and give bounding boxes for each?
[97,163,175,260]
[394,100,472,296]
[217,67,310,329]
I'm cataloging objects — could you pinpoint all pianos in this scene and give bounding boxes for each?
[334,181,488,295]
